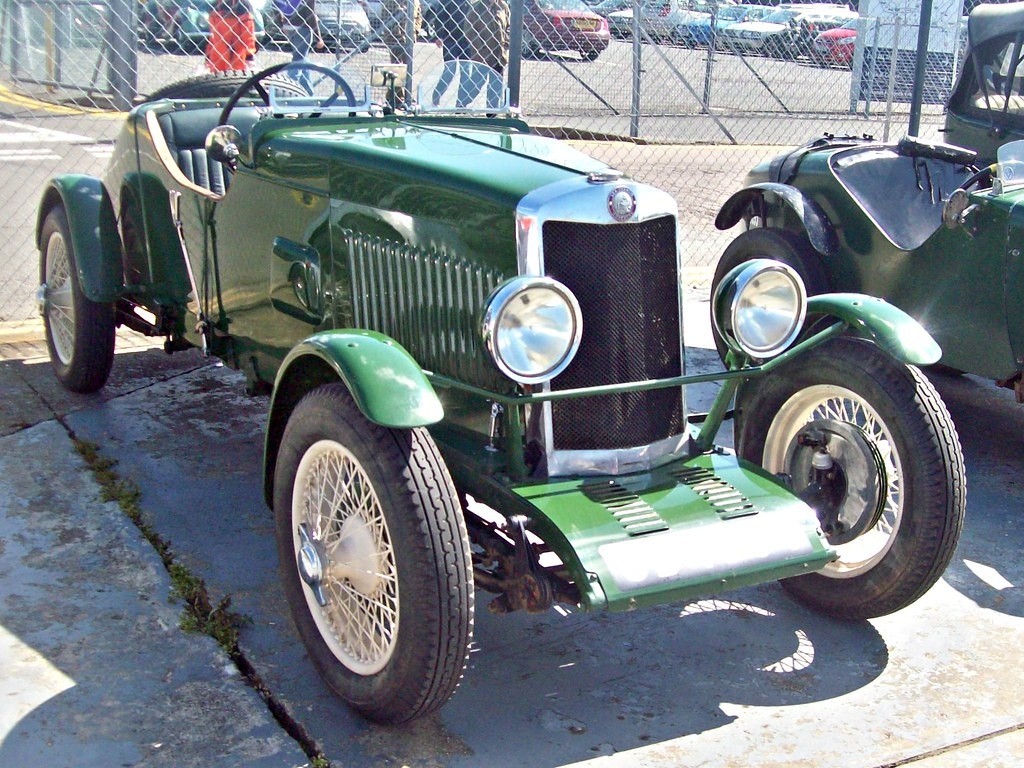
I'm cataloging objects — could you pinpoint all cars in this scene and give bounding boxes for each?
[422,1,610,61]
[590,0,967,82]
[16,0,383,53]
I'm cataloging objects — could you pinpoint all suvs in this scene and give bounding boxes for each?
[37,63,965,726]
[710,2,1023,405]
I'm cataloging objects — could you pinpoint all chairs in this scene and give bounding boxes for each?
[835,145,983,250]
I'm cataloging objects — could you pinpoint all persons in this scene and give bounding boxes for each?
[433,0,475,106]
[381,0,422,108]
[457,0,511,117]
[205,0,254,75]
[273,0,323,96]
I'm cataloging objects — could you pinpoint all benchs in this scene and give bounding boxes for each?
[157,102,389,196]
